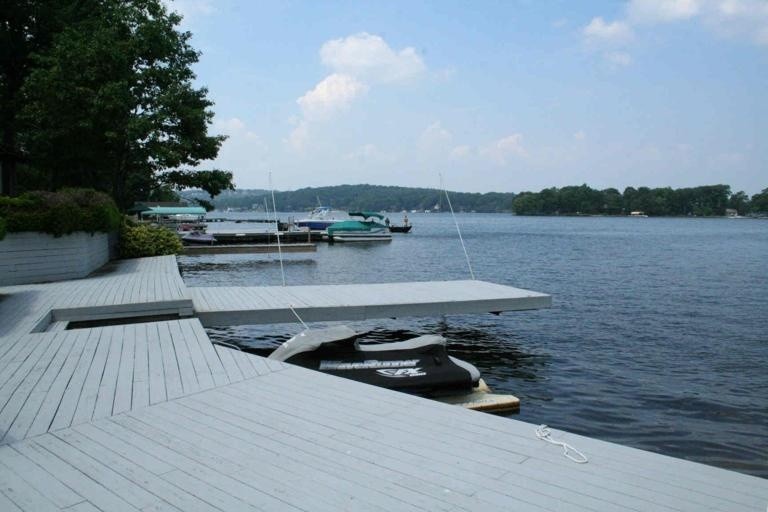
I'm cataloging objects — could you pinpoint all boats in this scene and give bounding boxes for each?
[266,324,522,415]
[321,214,393,241]
[182,230,218,245]
[385,224,412,234]
[275,206,380,229]
[177,222,206,235]
